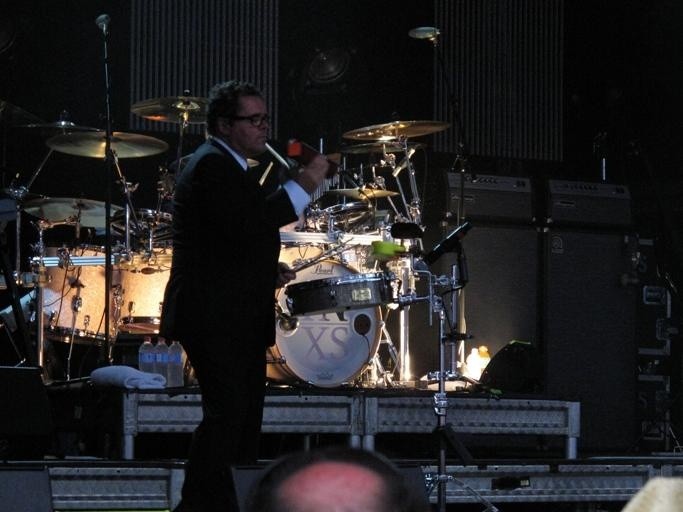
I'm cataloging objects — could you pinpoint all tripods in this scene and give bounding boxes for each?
[426,312,496,511]
[421,265,500,395]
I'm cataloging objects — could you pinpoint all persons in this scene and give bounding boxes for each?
[158,79,331,512]
[244,447,425,512]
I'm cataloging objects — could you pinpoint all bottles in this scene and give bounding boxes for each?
[461,345,491,381]
[138,335,188,389]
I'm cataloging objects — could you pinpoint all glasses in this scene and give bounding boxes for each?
[229,111,271,127]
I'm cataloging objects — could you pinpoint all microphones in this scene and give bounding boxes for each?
[92,13,112,27]
[425,222,474,262]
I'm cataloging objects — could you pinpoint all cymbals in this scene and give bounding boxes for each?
[25,198,121,229]
[132,97,212,125]
[343,120,445,139]
[325,188,398,200]
[49,131,168,159]
[340,143,403,154]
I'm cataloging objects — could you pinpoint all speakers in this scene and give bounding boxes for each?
[407,224,543,397]
[542,233,644,452]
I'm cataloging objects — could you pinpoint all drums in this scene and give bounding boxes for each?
[267,246,384,388]
[113,245,171,333]
[285,273,383,315]
[41,245,119,344]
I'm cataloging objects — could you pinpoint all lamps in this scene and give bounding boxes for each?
[408,26,470,156]
[300,37,359,96]
